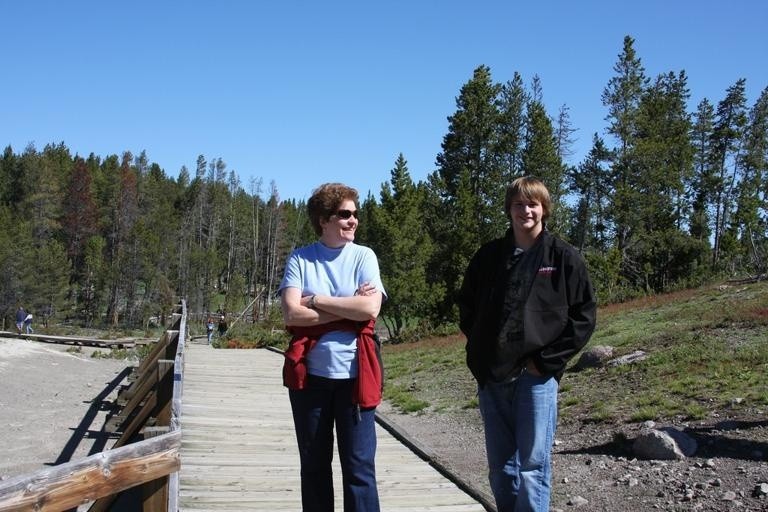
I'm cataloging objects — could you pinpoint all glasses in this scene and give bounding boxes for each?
[332,210,359,220]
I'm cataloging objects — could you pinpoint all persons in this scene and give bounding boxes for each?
[457,177,596,512]
[24,310,33,335]
[206,318,214,344]
[217,315,226,337]
[274,183,387,508]
[16,306,26,334]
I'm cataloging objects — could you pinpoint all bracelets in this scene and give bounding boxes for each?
[307,293,315,309]
